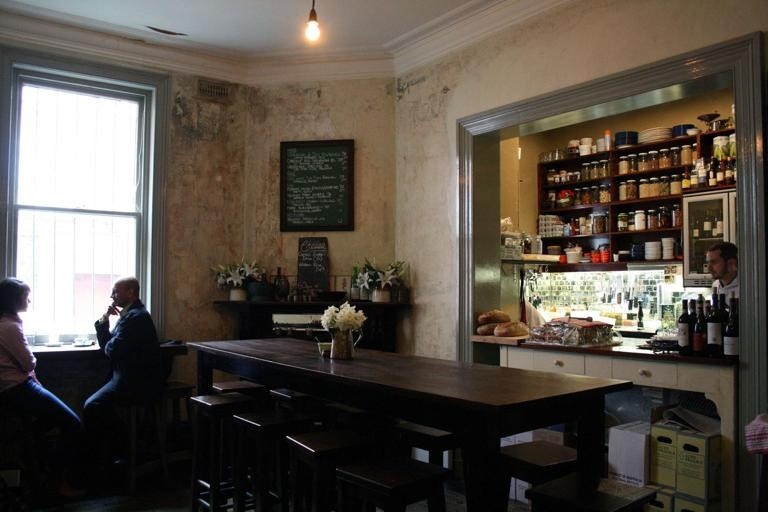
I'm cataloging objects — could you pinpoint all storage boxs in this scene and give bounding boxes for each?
[646,420,721,512]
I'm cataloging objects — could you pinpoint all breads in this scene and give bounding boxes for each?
[476,310,613,346]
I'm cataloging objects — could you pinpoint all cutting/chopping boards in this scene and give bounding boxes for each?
[471,333,529,348]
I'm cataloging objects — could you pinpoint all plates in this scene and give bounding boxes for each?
[645,237,675,261]
[696,113,721,122]
[540,124,700,163]
[617,133,737,239]
[547,244,610,264]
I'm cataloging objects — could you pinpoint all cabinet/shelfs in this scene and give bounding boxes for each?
[537,128,736,288]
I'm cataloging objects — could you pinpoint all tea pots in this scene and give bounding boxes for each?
[328,326,363,359]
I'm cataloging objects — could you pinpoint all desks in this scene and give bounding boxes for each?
[214,299,413,353]
[187,340,633,512]
[0,346,187,503]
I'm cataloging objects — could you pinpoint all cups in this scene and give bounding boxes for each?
[317,343,332,358]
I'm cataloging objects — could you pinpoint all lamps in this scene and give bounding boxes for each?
[305,0,320,41]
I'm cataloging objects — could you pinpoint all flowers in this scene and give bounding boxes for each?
[211,255,263,289]
[356,255,409,292]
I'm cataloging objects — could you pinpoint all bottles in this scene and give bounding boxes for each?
[677,287,739,360]
[619,243,644,261]
[350,264,372,301]
[261,266,282,300]
[724,113,735,129]
[521,232,542,255]
[564,285,639,329]
[538,159,609,236]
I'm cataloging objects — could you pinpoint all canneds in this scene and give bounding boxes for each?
[546,143,699,234]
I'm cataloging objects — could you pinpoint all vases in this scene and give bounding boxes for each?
[229,289,247,301]
[372,290,390,303]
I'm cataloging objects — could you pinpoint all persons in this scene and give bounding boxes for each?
[0,276,88,498]
[82,277,168,471]
[704,240,738,310]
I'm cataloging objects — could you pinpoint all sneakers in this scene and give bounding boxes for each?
[54,478,91,500]
